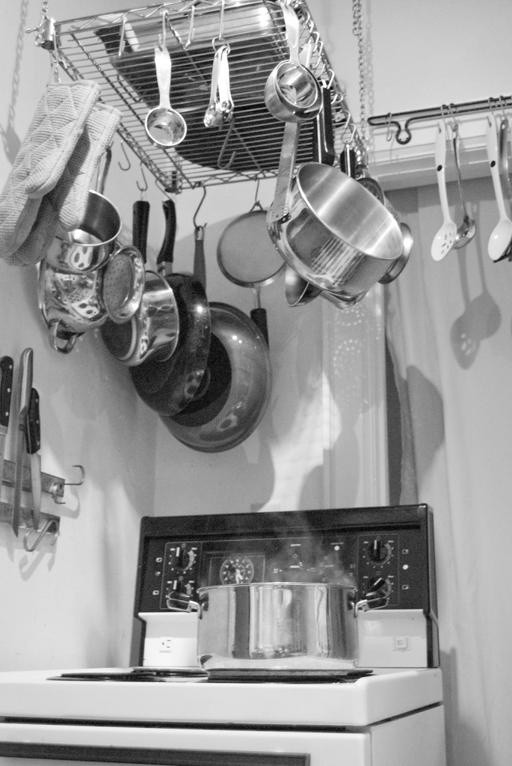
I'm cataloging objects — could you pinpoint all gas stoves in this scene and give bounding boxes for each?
[45,502,441,686]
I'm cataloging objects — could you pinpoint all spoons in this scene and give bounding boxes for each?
[451,123,477,249]
[144,45,187,149]
[429,122,457,262]
[485,113,512,262]
[203,46,235,127]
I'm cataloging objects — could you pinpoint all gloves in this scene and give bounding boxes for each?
[6,103,122,266]
[0,82,103,253]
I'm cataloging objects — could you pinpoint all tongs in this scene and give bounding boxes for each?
[8,345,44,536]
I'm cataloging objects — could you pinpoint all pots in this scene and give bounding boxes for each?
[130,199,212,419]
[101,200,180,367]
[161,226,274,454]
[196,581,361,675]
[267,58,413,307]
[43,187,122,275]
[38,146,146,355]
[217,209,286,357]
[263,2,325,123]
